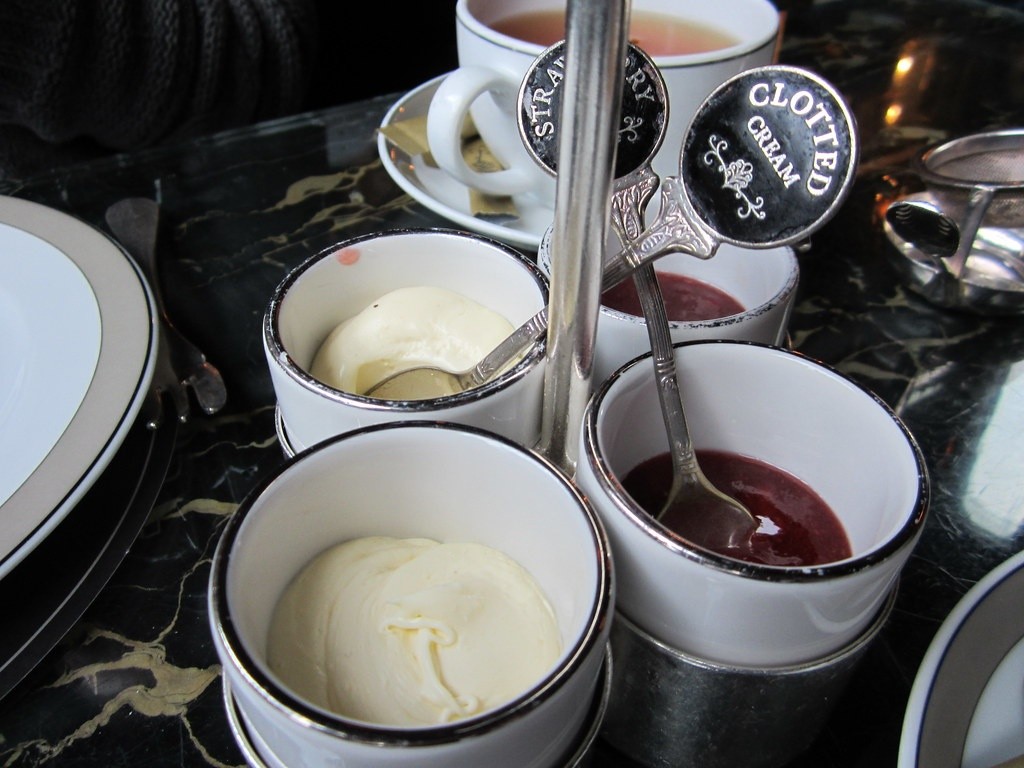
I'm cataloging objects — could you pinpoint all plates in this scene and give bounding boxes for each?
[221,642,613,768]
[0,188,161,581]
[896,548,1024,767]
[378,71,558,248]
[612,602,905,677]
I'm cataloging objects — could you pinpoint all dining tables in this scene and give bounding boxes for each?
[1,16,1023,768]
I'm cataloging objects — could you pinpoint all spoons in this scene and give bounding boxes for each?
[105,197,228,413]
[518,36,755,554]
[367,63,858,400]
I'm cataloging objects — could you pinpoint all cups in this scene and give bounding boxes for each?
[425,0,780,212]
[208,423,616,768]
[264,223,550,456]
[576,338,930,664]
